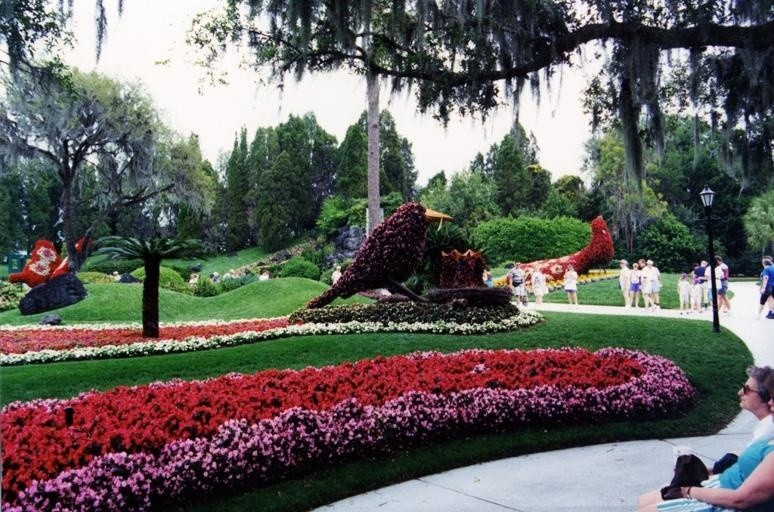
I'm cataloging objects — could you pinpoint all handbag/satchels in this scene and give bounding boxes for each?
[522,292,528,306]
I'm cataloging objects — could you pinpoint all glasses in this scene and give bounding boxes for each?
[741,383,759,394]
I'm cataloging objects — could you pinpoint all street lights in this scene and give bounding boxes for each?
[693,183,730,333]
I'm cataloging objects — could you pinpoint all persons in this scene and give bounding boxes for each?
[482,256,774,320]
[110,271,121,281]
[332,266,342,285]
[189,269,269,286]
